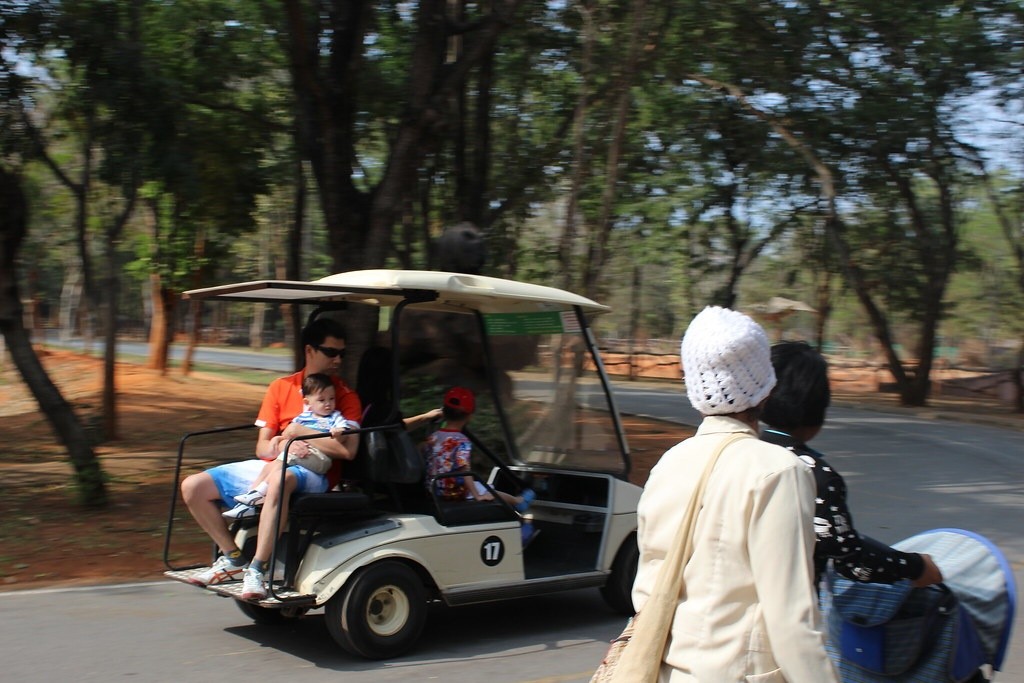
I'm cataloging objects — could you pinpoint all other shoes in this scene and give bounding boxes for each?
[521,524,534,540]
[516,488,534,511]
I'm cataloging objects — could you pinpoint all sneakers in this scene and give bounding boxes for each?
[234,490,267,507]
[241,566,267,599]
[221,502,255,519]
[189,556,250,584]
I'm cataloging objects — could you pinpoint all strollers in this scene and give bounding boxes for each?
[816,526,1016,683]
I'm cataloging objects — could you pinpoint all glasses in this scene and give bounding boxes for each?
[310,343,346,359]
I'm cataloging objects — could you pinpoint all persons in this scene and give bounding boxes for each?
[356,345,539,545]
[180,315,363,601]
[629,304,839,682]
[222,373,347,521]
[757,341,944,603]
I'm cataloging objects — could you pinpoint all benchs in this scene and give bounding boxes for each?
[286,337,513,529]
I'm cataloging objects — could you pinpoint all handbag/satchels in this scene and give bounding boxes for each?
[590,605,675,682]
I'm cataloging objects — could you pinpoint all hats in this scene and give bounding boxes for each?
[445,387,476,413]
[680,305,776,415]
